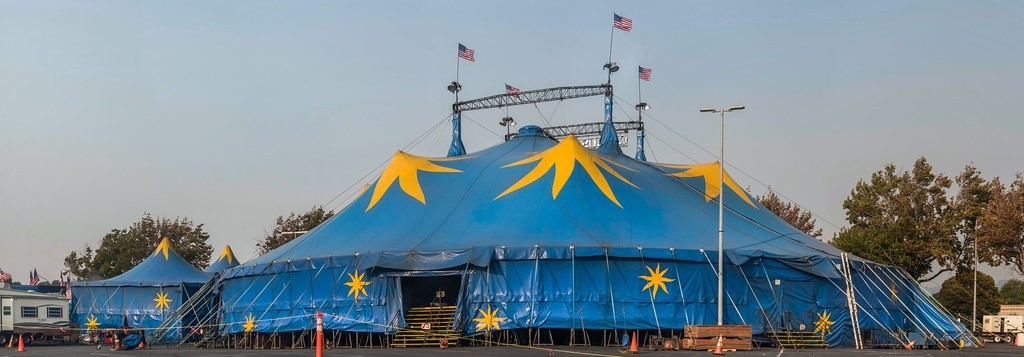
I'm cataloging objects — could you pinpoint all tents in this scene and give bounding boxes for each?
[70,123,981,348]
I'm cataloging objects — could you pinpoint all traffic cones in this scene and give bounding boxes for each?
[6,335,14,348]
[629,331,639,352]
[15,334,27,352]
[713,334,725,355]
[906,339,915,349]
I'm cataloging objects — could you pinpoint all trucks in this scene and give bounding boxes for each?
[982,314,1023,343]
[0,287,70,345]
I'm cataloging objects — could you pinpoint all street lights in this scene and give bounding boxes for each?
[700,105,746,326]
